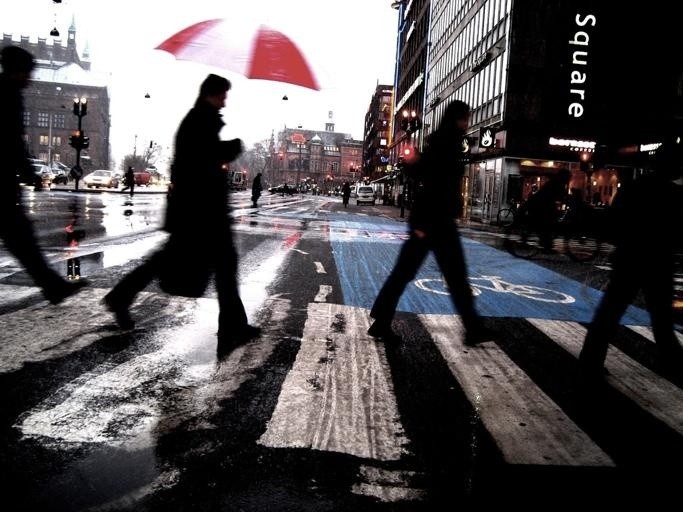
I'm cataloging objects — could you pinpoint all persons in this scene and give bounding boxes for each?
[250,172,263,207]
[365,97,500,344]
[524,170,575,249]
[119,165,135,197]
[342,181,350,210]
[0,44,91,307]
[573,142,683,388]
[100,74,260,344]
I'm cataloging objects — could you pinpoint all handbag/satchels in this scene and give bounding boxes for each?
[159,264,211,298]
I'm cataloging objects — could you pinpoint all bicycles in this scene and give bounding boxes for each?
[498,197,521,227]
[503,203,600,262]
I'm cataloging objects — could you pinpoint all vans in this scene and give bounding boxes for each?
[357,186,375,205]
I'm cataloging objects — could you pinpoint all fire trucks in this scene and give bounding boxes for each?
[227,171,247,191]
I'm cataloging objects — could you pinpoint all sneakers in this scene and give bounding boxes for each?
[102,295,135,329]
[219,325,260,336]
[462,328,498,345]
[368,320,401,338]
[47,279,88,305]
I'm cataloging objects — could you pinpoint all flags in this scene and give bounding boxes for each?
[156,18,318,94]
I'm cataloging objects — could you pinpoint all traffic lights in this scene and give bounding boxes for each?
[68,130,90,150]
[401,110,409,130]
[404,148,411,156]
[411,110,418,131]
[73,98,80,116]
[80,98,87,116]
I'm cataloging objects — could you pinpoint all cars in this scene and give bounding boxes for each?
[268,184,298,194]
[86,170,119,188]
[26,158,67,185]
[123,172,150,187]
